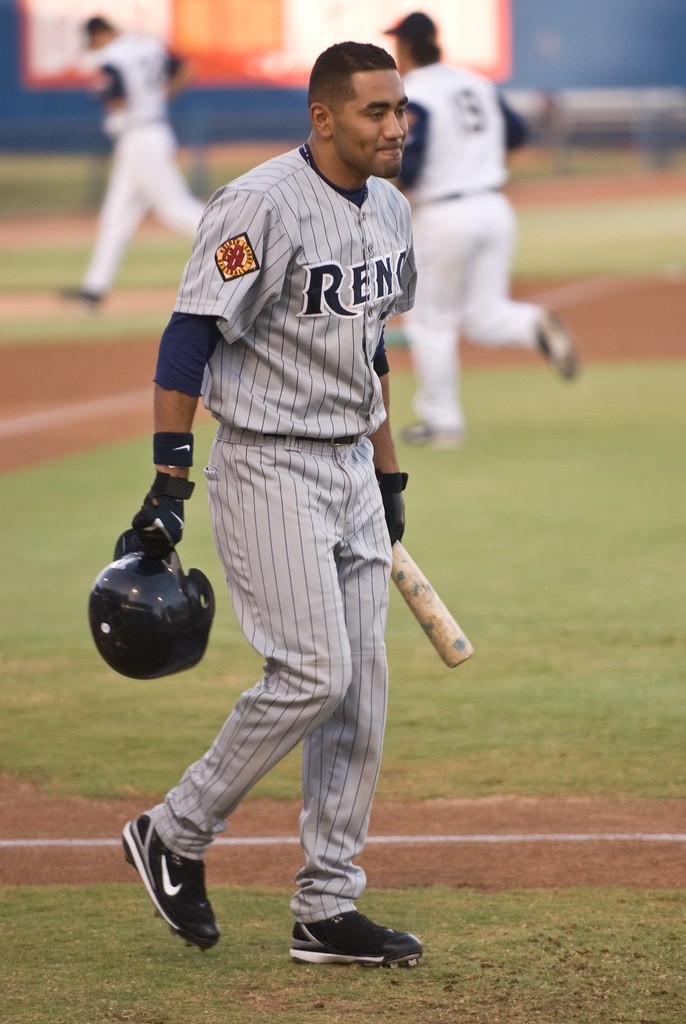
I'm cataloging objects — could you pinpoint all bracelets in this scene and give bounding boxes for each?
[152,431,193,468]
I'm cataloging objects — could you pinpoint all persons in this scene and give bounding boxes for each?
[63,15,204,301]
[120,40,426,967]
[385,13,580,445]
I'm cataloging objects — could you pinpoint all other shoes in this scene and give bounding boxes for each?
[533,310,577,382]
[403,423,435,443]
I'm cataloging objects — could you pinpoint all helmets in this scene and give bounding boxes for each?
[87,528,216,680]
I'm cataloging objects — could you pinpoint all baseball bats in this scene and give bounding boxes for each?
[389,540,475,669]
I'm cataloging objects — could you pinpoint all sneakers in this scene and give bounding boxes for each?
[120,813,221,949]
[289,911,423,969]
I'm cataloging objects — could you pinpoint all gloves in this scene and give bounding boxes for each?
[131,471,195,562]
[374,468,408,547]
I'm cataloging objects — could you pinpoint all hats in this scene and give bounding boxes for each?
[85,18,103,29]
[385,12,436,35]
